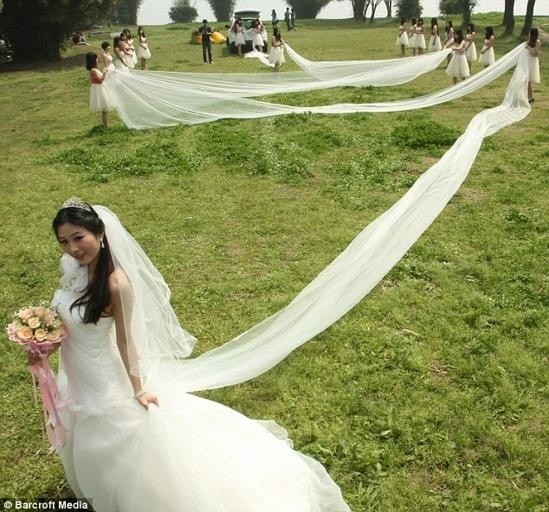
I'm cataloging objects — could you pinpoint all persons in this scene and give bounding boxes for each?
[73,26,152,128]
[30,200,350,512]
[511,28,541,109]
[271,6,297,34]
[398,16,496,92]
[235,19,246,56]
[252,20,265,53]
[199,19,215,65]
[272,28,286,70]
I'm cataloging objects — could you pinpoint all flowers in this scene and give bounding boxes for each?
[5,300,69,362]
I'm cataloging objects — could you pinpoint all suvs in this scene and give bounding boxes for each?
[224,8,268,53]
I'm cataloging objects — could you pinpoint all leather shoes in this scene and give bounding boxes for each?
[528,98,535,105]
